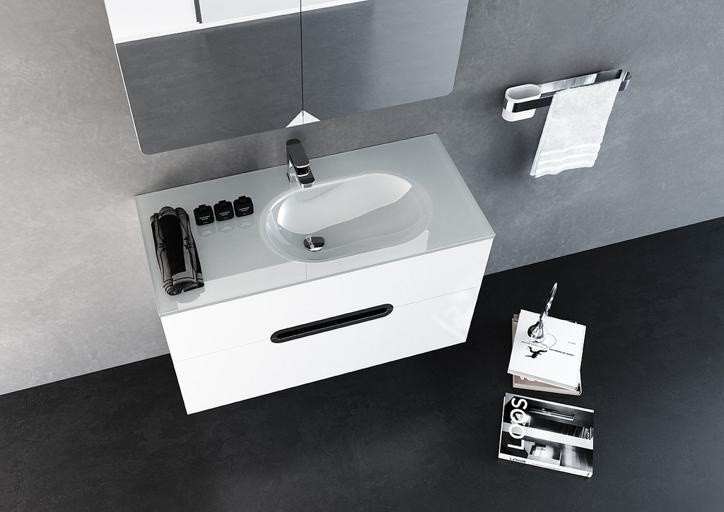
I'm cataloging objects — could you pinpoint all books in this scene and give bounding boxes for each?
[507,309,586,396]
[498,393,594,480]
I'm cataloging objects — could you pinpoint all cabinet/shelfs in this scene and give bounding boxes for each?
[134,133,496,416]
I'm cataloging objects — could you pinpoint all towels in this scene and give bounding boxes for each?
[150,206,205,296]
[528,77,623,180]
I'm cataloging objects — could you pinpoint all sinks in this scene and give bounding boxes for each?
[260,170,431,262]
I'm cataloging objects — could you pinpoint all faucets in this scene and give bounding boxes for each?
[286,137,316,190]
